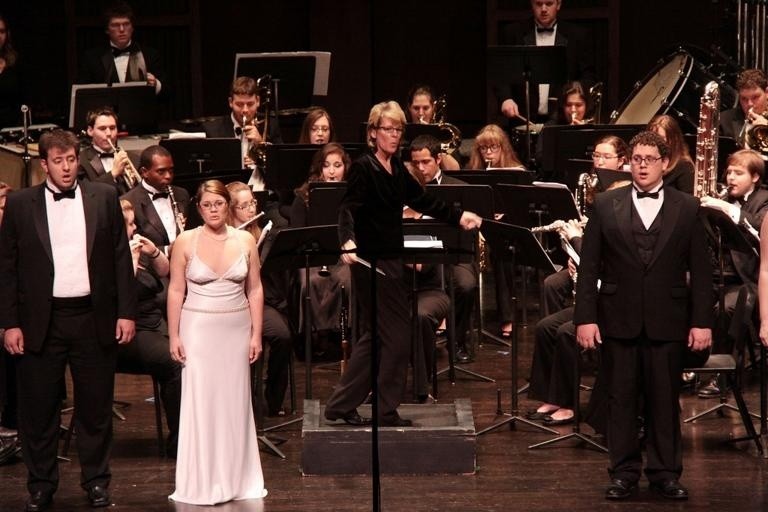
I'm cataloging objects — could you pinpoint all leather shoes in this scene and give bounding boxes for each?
[24,487,53,512]
[456,349,474,365]
[606,475,640,499]
[525,407,560,421]
[543,410,581,426]
[323,405,373,426]
[696,373,732,400]
[375,410,412,428]
[645,478,689,499]
[80,483,112,509]
[497,323,515,340]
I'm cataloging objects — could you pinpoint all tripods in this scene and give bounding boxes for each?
[682,321,763,424]
[432,260,511,384]
[61,355,132,424]
[251,333,289,457]
[527,336,607,454]
[478,328,558,438]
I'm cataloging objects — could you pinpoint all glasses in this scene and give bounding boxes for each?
[630,154,663,165]
[231,199,257,211]
[376,125,405,135]
[198,200,227,211]
[478,143,502,154]
[591,152,618,160]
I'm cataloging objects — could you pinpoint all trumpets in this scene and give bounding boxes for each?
[108,138,142,190]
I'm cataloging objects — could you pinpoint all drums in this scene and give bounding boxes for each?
[610,50,738,139]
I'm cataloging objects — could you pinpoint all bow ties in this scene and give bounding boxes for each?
[536,25,554,33]
[425,175,441,186]
[148,189,170,201]
[632,184,662,200]
[110,45,134,58]
[44,182,78,201]
[235,126,243,136]
[726,194,749,206]
[94,148,115,159]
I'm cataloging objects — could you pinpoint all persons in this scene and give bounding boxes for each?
[489,1,583,130]
[0,127,139,512]
[165,179,267,503]
[571,131,719,500]
[1,75,768,443]
[0,18,25,128]
[68,6,163,120]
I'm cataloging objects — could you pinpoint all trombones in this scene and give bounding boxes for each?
[240,76,271,170]
[419,95,461,156]
